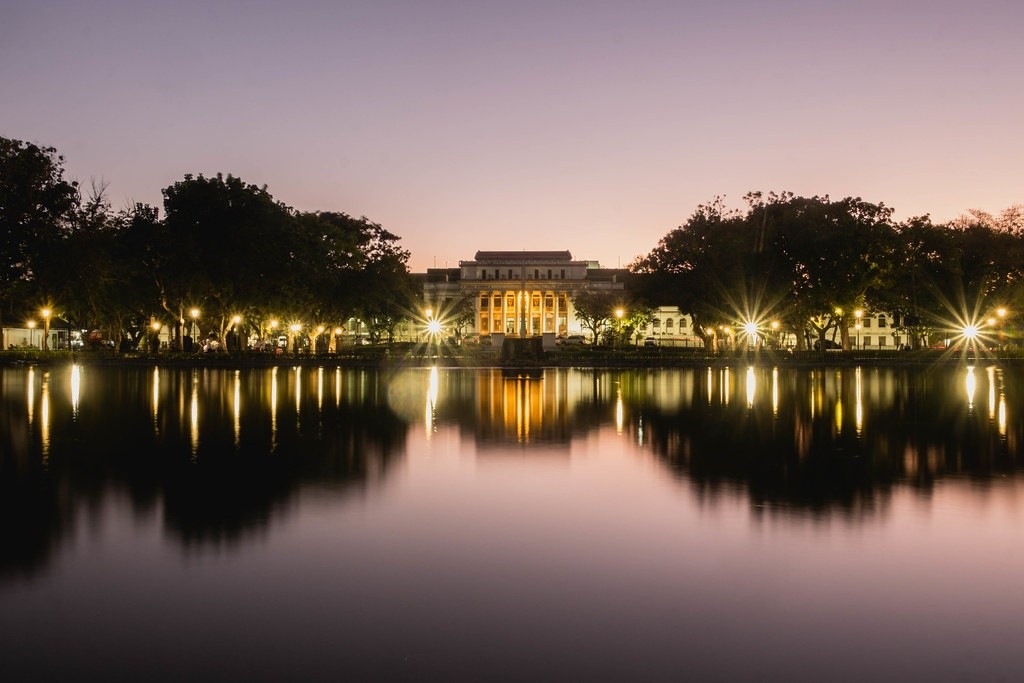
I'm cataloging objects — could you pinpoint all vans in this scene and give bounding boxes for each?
[278,336,287,348]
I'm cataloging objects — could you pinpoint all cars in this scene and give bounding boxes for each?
[356,338,374,346]
[813,338,842,351]
[560,334,592,345]
[643,337,657,347]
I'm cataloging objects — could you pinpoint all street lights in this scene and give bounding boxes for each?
[291,326,298,354]
[234,316,241,352]
[272,320,276,353]
[28,322,34,348]
[988,318,996,353]
[192,309,199,349]
[43,308,49,352]
[707,329,714,356]
[998,309,1004,353]
[856,310,861,349]
[616,309,624,350]
